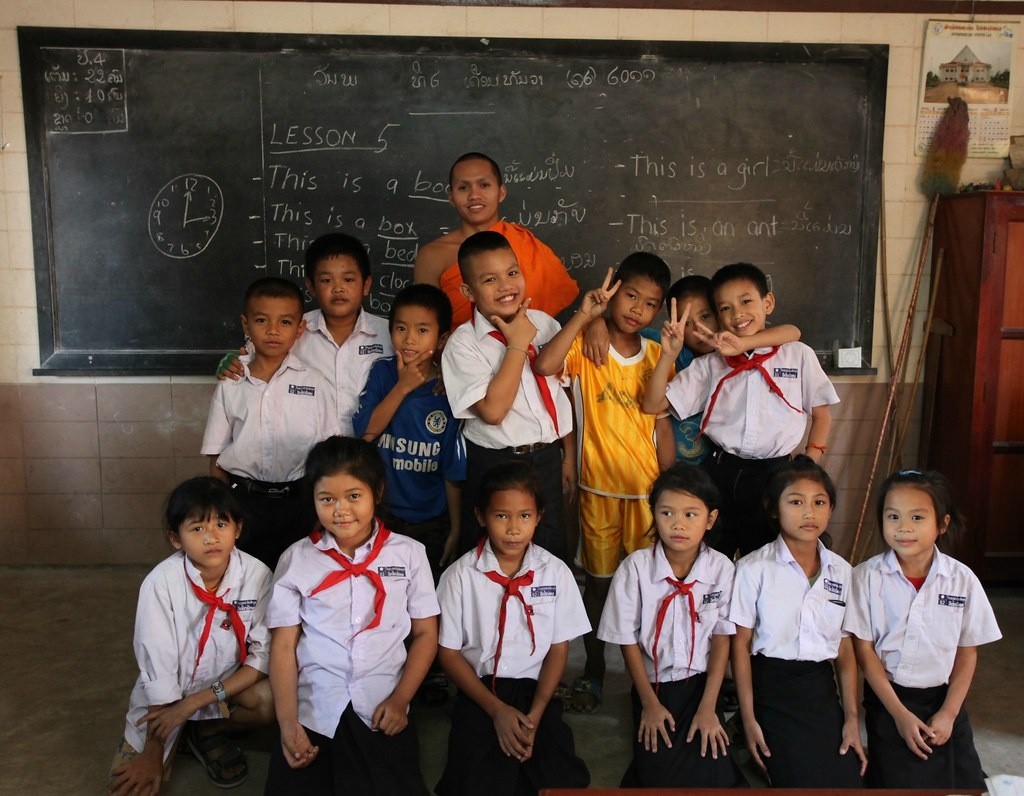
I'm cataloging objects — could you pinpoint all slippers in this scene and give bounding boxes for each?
[549,682,574,711]
[574,676,603,714]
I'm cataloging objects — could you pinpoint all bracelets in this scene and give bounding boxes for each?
[804,444,826,455]
[505,345,528,354]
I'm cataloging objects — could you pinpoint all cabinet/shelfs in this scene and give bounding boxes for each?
[929,193,1024,587]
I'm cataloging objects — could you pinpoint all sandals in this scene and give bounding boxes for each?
[176,722,249,788]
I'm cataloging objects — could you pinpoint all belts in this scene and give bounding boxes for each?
[465,437,561,456]
[230,475,309,500]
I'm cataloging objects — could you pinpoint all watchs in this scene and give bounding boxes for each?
[210,681,226,702]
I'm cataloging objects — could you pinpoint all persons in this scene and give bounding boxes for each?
[105,151,1002,697]
[107,475,275,796]
[433,462,595,795]
[596,460,750,788]
[728,453,868,789]
[264,432,441,796]
[841,465,1003,789]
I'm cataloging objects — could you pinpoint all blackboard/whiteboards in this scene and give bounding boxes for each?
[16,27,889,376]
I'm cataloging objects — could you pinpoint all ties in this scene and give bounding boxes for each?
[183,557,247,683]
[307,516,391,644]
[652,542,697,697]
[693,345,804,443]
[470,313,561,439]
[477,535,536,696]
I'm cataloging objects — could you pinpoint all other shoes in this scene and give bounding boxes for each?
[732,731,752,767]
[721,678,739,711]
